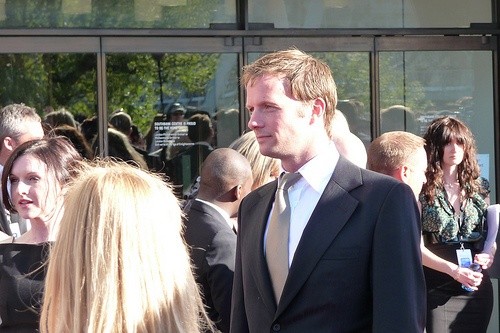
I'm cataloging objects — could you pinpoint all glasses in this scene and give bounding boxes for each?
[172,112,184,116]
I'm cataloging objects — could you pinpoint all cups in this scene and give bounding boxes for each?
[461,264,481,292]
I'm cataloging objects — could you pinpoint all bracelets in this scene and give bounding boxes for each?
[454,266,460,280]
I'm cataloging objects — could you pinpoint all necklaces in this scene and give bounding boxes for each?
[444,180,453,188]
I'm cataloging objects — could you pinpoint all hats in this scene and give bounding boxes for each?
[170,103,186,112]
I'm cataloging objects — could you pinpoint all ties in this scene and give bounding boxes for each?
[264,172,302,307]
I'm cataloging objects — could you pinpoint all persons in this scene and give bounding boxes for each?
[43,98,416,196]
[0,102,494,333]
[229,46,427,333]
[37,155,219,333]
